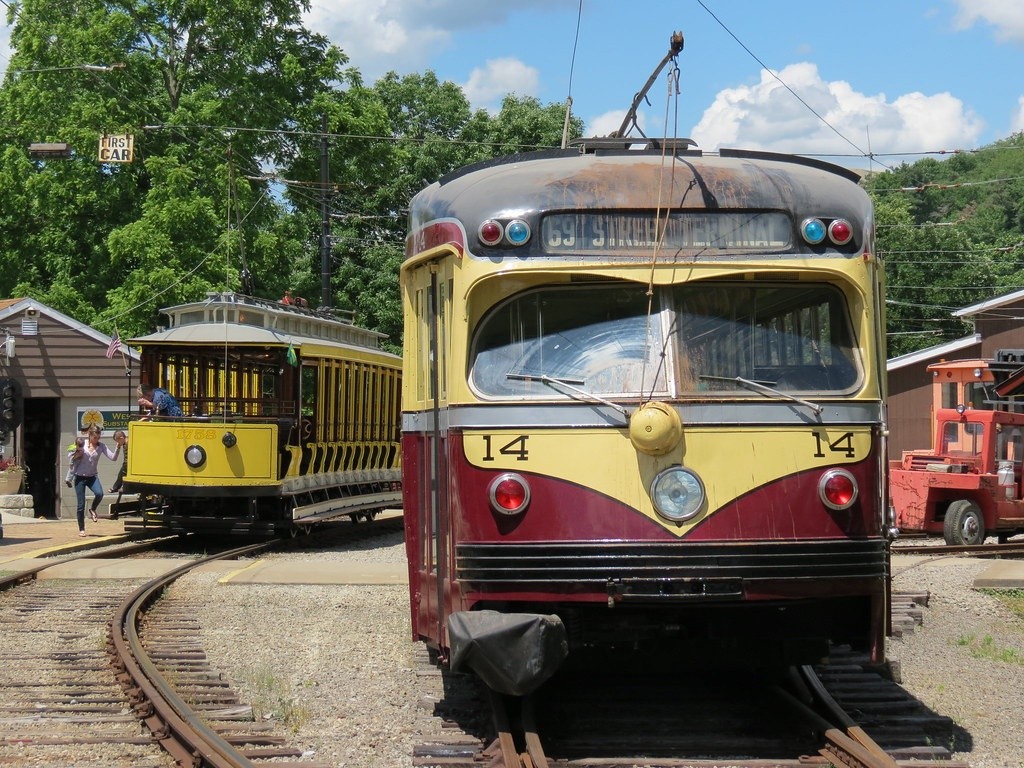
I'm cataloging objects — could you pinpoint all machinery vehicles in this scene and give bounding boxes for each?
[888,348,1024,547]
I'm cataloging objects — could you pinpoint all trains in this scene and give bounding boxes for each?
[396,133,897,695]
[109,294,406,537]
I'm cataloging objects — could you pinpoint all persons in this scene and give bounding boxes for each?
[65,437,85,488]
[70,426,121,537]
[107,430,128,493]
[137,384,184,423]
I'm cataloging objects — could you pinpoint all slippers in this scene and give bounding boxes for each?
[89,509,97,522]
[79,532,86,536]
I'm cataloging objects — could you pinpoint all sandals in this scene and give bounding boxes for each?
[108,488,118,493]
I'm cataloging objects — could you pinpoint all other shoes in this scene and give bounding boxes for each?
[66,480,72,488]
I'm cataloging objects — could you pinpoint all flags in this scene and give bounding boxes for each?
[106,328,121,358]
